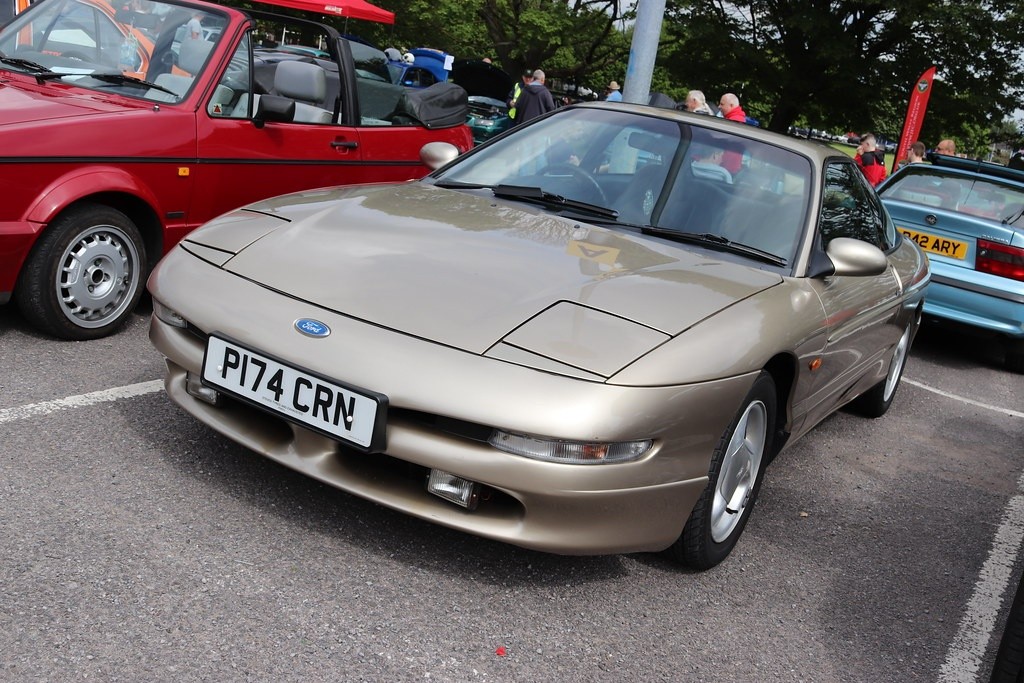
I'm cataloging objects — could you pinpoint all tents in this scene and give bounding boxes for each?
[252,0,394,50]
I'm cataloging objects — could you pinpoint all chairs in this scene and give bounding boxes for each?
[611,157,677,229]
[718,186,783,254]
[232,60,336,122]
[141,38,234,116]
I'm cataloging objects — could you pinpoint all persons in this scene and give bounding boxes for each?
[504,68,622,176]
[183,13,204,42]
[683,90,747,184]
[852,133,961,189]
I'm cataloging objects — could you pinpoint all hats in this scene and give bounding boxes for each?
[607,81,620,89]
[523,69,534,78]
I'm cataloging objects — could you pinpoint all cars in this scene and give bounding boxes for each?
[0,0,194,81]
[391,48,455,90]
[549,91,584,108]
[0,0,473,340]
[842,152,1024,374]
[465,96,513,146]
[279,44,331,58]
[788,126,861,144]
[155,24,247,56]
[563,80,598,99]
[885,143,898,153]
[636,116,786,197]
[147,101,931,572]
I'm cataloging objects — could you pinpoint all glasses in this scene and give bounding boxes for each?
[937,146,948,150]
